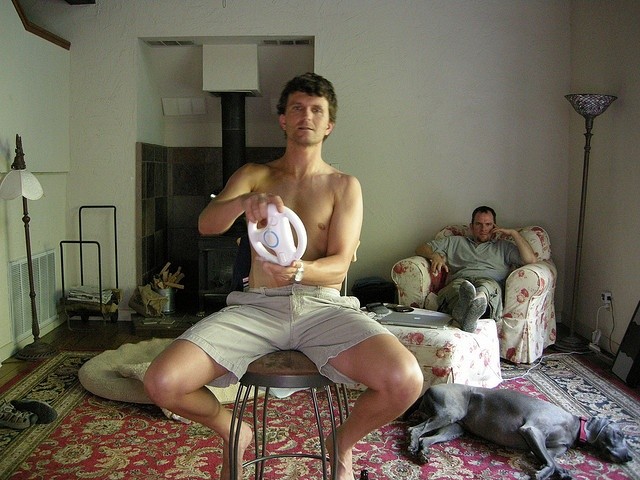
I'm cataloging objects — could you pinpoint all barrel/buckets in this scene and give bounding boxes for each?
[158,288,174,312]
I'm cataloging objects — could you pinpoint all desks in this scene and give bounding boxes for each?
[360,302,504,398]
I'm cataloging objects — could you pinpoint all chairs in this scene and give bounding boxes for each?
[228,269,351,480]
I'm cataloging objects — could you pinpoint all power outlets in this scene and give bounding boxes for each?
[601,292,611,303]
[605,303,611,311]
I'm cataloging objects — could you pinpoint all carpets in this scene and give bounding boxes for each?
[1,342,640,476]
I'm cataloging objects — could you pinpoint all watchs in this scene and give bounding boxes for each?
[294,263,304,283]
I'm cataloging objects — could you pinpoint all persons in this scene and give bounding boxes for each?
[144,73,425,480]
[416,205,536,332]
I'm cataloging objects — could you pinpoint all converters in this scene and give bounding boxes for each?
[600,290,611,305]
[588,342,601,354]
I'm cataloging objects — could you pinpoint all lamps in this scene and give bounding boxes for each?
[553,94,618,352]
[0,134,59,362]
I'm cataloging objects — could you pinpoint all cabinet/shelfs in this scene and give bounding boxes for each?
[198,236,248,317]
[169,146,222,261]
[136,142,168,285]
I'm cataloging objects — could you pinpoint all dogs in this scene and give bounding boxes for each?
[401,382,633,480]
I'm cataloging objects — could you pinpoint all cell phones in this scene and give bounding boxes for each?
[394,305,413,312]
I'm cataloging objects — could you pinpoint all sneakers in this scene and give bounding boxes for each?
[0,400,38,431]
[11,397,58,424]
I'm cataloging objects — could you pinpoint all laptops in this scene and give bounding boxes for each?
[381,311,453,329]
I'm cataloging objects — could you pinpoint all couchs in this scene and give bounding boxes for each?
[390,225,558,365]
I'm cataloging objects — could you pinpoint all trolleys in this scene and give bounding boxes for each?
[60,206,118,323]
